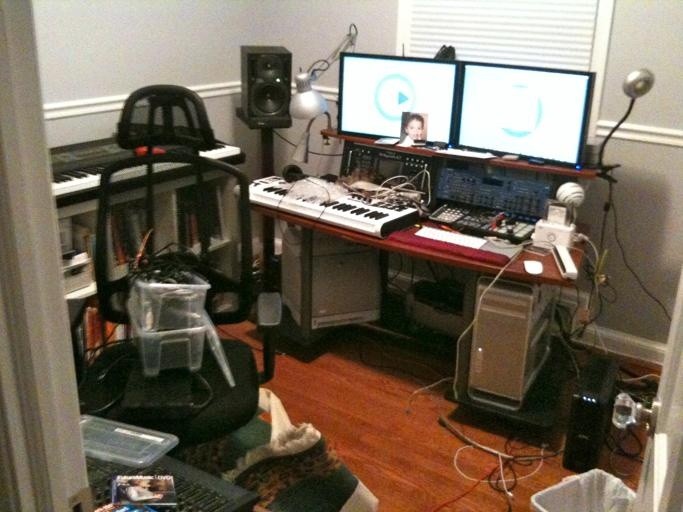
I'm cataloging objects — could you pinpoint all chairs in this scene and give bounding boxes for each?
[78,76,279,463]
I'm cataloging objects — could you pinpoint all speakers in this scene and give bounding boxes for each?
[241,45,292,118]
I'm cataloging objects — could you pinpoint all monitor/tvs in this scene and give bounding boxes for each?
[453,59,597,168]
[337,51,461,147]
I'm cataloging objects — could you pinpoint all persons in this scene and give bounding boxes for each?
[126,479,164,503]
[402,114,425,146]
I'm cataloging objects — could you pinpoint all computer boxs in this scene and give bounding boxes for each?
[466,271,554,410]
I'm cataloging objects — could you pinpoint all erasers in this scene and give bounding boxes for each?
[503,155,518,161]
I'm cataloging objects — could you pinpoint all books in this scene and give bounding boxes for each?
[94,503,157,512]
[74,183,226,364]
[112,473,179,506]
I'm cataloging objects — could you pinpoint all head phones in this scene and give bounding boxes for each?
[282,163,338,182]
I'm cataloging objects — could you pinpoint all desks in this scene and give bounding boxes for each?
[246,126,611,438]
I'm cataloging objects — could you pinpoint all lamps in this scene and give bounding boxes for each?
[597,68,656,169]
[289,109,337,166]
[287,21,357,123]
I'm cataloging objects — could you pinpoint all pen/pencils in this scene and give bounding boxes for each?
[115,427,165,444]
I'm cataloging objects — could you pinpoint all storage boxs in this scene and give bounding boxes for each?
[125,266,213,332]
[123,300,209,375]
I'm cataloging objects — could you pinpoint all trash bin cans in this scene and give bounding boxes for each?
[530,469,636,512]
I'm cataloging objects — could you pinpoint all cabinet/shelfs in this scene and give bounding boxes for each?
[57,166,250,359]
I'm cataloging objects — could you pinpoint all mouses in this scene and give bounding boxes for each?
[523,258,545,277]
